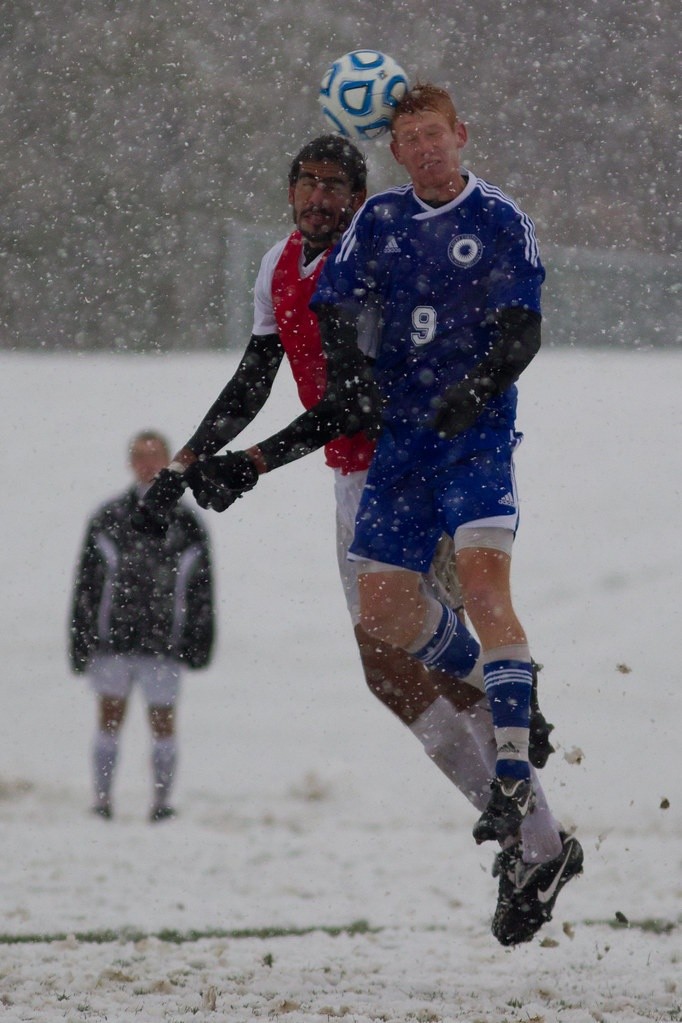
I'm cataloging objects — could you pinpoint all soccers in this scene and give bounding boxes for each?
[318,50,409,138]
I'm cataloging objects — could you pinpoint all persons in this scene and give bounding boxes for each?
[129,136,582,945]
[67,432,217,823]
[312,85,557,842]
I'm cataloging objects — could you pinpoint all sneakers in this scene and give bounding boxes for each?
[473,778,537,842]
[526,657,553,768]
[491,832,583,946]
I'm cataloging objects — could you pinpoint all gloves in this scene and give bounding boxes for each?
[434,376,500,435]
[335,370,380,438]
[130,466,185,539]
[184,450,258,513]
